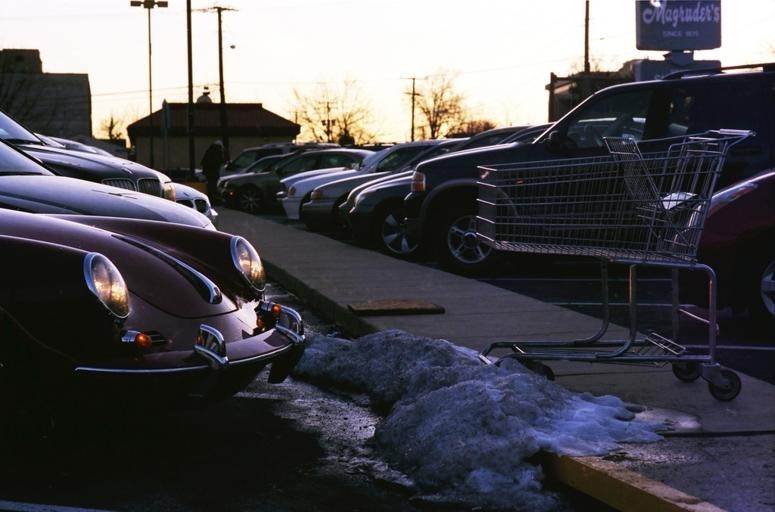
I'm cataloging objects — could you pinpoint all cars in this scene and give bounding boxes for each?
[221,62,775,331]
[1,207,308,442]
[2,110,221,233]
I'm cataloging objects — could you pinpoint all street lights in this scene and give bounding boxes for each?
[219,43,236,167]
[130,0,169,168]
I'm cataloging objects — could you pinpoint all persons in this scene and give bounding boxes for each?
[203,140,224,208]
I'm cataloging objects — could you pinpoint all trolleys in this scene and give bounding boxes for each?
[471,123,755,403]
